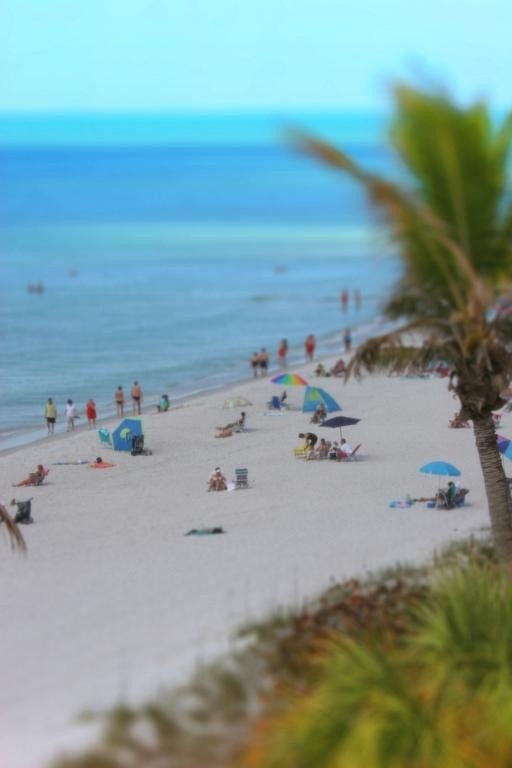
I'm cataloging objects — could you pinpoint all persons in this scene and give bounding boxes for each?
[313,358,348,379]
[41,397,57,436]
[305,438,329,462]
[248,351,258,377]
[129,380,144,417]
[84,399,97,430]
[208,465,226,492]
[309,402,326,424]
[63,398,78,433]
[341,328,351,352]
[276,338,288,369]
[297,432,318,451]
[9,462,45,488]
[252,347,269,376]
[214,412,246,431]
[155,393,170,412]
[113,384,125,418]
[439,479,463,509]
[303,334,315,363]
[330,438,353,462]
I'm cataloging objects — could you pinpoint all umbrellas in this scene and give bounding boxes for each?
[218,395,253,410]
[108,417,143,453]
[418,460,462,480]
[302,386,343,415]
[268,372,308,390]
[316,414,361,437]
